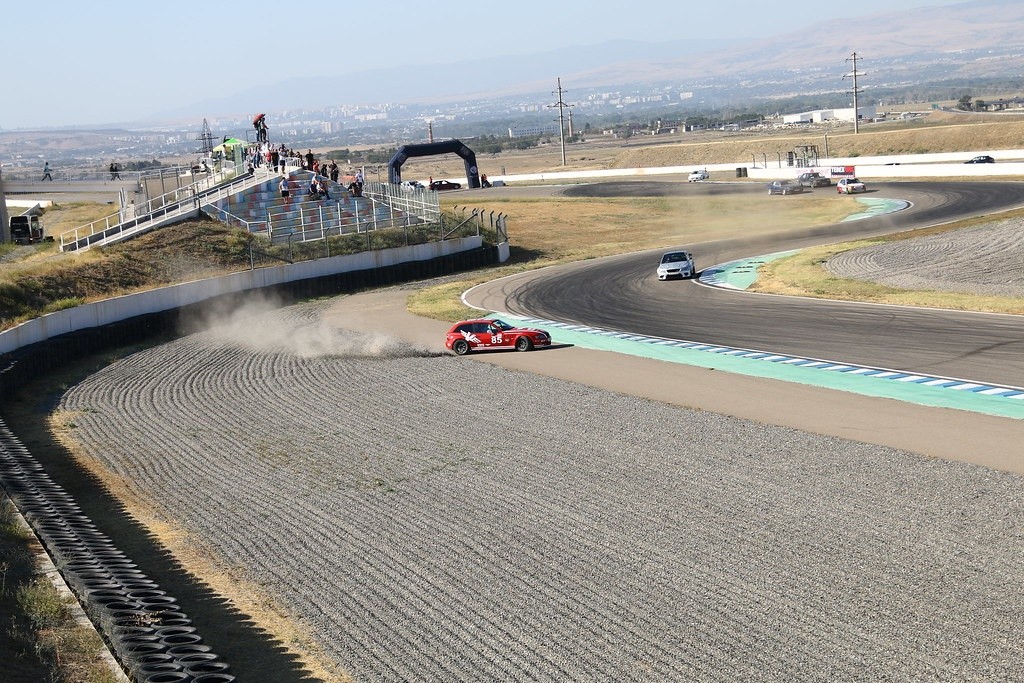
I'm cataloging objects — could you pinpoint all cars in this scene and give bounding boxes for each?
[445,319,551,355]
[797,172,831,188]
[837,177,866,195]
[429,180,461,190]
[768,180,803,195]
[657,251,695,280]
[400,181,425,195]
[688,170,707,182]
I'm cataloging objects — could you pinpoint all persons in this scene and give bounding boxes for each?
[41,162,52,182]
[481,174,487,188]
[428,177,432,188]
[222,114,365,205]
[110,164,121,181]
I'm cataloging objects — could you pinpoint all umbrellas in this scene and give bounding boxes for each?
[213,138,252,151]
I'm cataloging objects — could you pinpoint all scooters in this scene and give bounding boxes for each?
[190,159,211,175]
